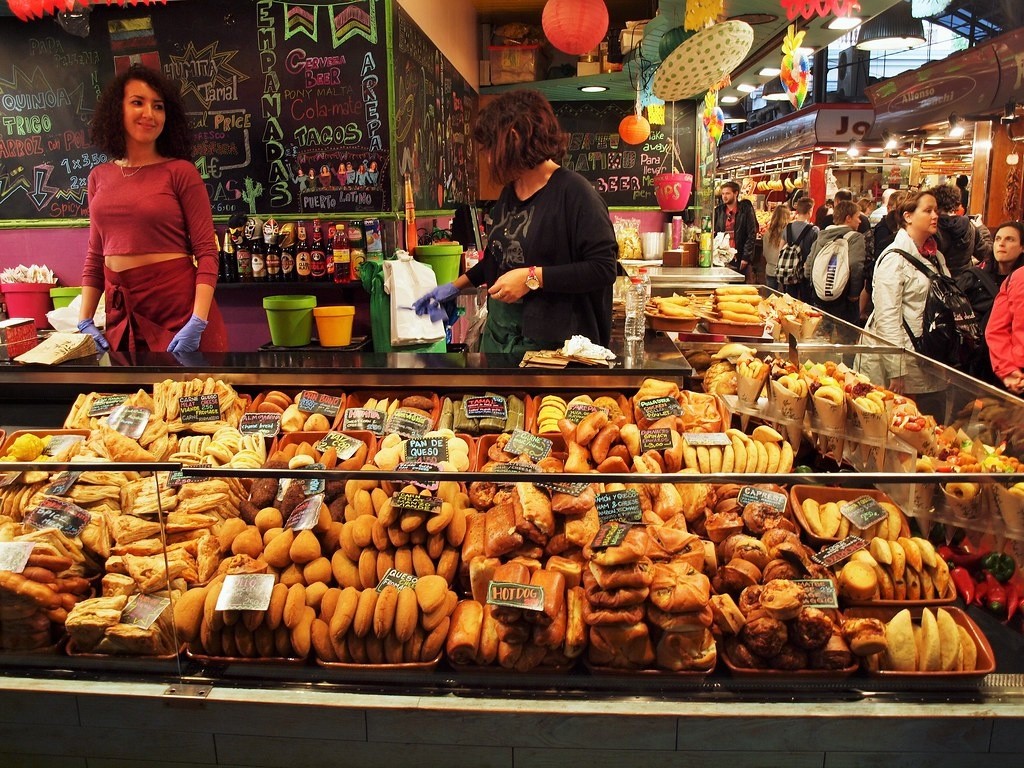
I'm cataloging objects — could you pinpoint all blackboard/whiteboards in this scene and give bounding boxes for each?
[546,99,698,211]
[392,0,483,219]
[0,0,392,219]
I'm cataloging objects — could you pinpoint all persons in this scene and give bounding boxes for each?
[714,181,757,284]
[76,61,229,353]
[956,175,969,214]
[413,87,619,352]
[852,185,1024,457]
[739,199,760,232]
[780,188,875,363]
[763,205,791,295]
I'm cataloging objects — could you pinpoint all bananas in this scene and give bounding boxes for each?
[757,177,804,191]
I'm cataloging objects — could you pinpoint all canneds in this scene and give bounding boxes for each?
[698,216,712,267]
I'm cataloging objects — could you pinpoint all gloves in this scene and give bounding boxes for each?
[77,320,108,352]
[172,353,205,368]
[414,283,459,317]
[166,316,208,353]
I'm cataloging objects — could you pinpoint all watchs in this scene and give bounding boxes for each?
[525,265,539,290]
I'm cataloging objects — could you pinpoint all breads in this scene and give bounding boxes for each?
[0,342,1022,676]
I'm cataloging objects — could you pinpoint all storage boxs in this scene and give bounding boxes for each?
[487,44,549,85]
[183,485,996,682]
[0,391,632,481]
[644,312,765,337]
[837,46,870,101]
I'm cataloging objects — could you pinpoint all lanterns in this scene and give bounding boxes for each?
[542,0,609,56]
[619,114,650,144]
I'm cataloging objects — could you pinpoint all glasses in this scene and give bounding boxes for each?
[727,211,732,221]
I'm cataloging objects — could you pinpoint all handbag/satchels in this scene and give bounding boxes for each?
[956,266,999,328]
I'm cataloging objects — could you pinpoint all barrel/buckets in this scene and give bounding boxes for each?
[312,305,356,347]
[49,287,82,309]
[413,245,464,287]
[0,282,56,329]
[263,295,316,347]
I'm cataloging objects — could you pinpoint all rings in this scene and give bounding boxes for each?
[894,389,897,393]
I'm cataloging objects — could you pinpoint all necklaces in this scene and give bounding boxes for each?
[120,154,152,178]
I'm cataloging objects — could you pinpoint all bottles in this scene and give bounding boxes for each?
[624,277,647,342]
[214,219,367,284]
[637,268,651,304]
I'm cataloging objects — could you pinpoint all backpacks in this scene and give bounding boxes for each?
[775,221,814,284]
[813,229,858,302]
[875,250,982,373]
